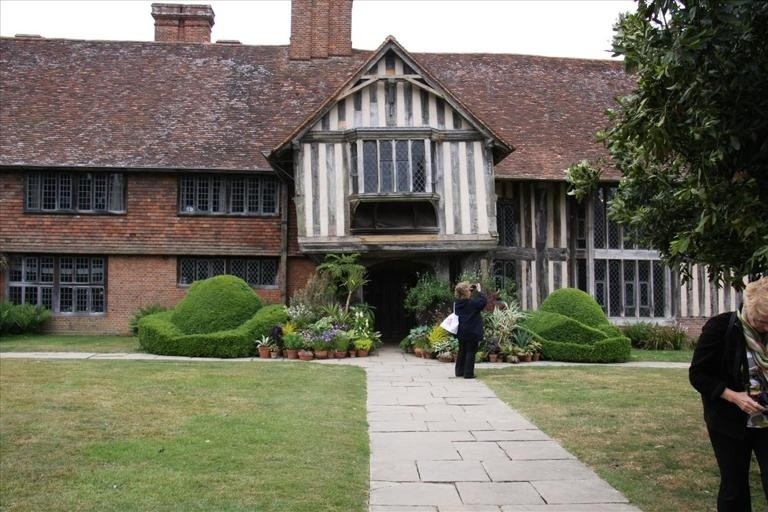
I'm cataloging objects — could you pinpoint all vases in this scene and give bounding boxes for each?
[256,345,371,361]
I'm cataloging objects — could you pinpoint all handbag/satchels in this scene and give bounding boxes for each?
[439,301,459,335]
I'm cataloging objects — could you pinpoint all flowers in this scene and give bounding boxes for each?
[398,300,546,364]
[256,299,380,351]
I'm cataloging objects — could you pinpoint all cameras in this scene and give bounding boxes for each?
[751,392,768,408]
[471,285,477,288]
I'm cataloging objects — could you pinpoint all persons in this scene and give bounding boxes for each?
[451,280,487,379]
[689,276,768,512]
